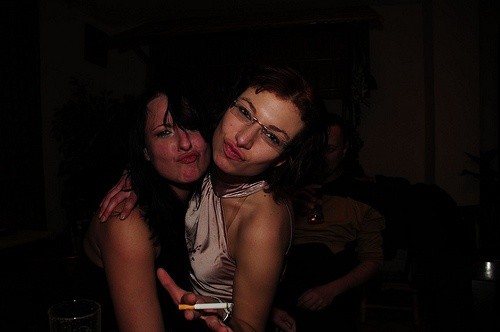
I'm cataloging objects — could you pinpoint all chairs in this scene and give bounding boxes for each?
[359,234,424,327]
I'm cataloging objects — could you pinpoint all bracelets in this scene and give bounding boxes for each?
[212,297,233,324]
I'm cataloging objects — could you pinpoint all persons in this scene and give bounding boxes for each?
[263,112,389,332]
[98,64,330,332]
[74,74,296,332]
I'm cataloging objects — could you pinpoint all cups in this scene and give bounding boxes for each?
[48,299,102,332]
[303,188,324,225]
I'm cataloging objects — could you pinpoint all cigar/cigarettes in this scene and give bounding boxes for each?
[177,302,233,311]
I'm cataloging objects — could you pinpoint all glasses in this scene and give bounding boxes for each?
[230,97,293,153]
